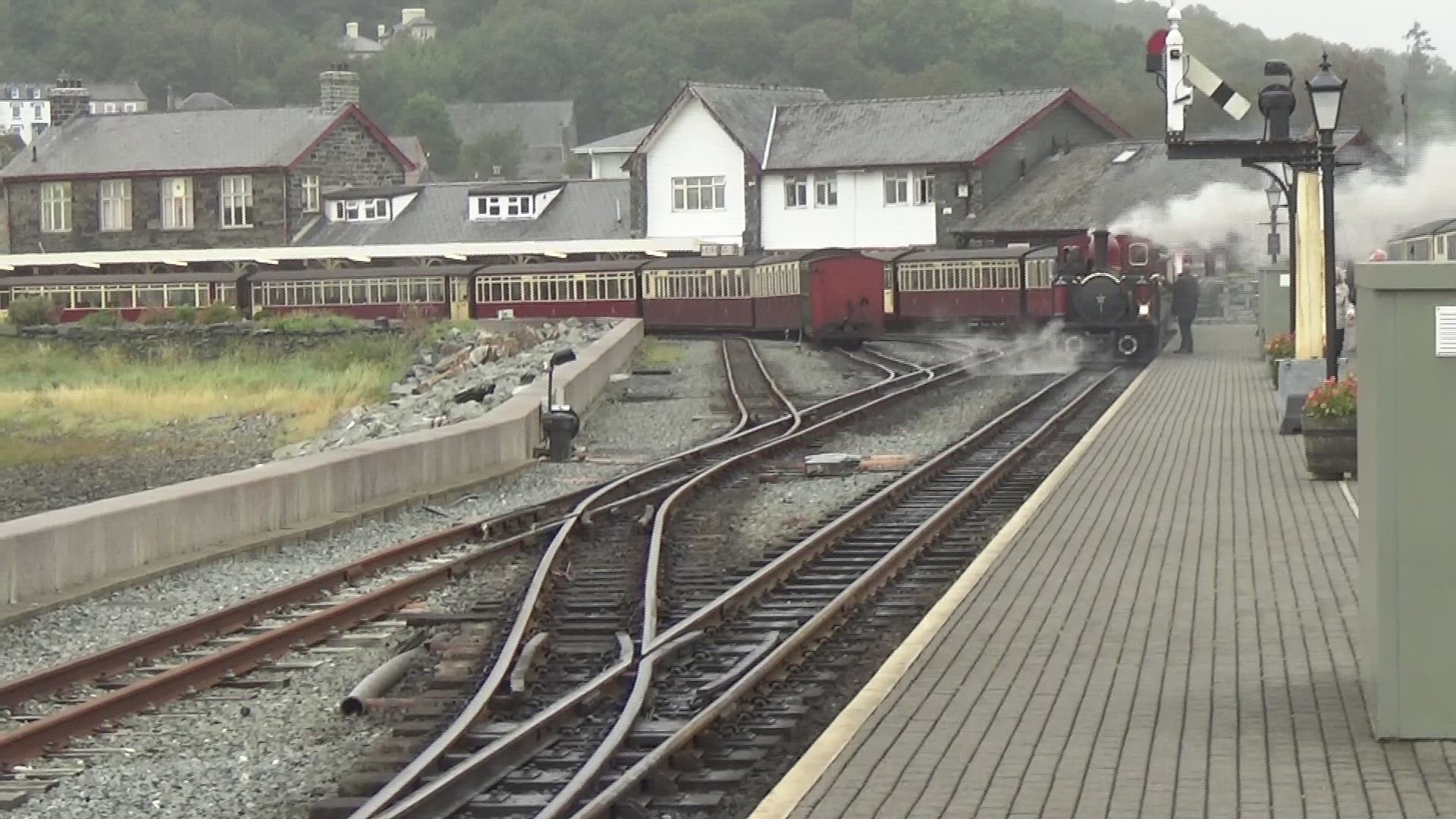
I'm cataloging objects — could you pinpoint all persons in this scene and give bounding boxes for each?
[1166,266,1199,353]
[1336,267,1355,356]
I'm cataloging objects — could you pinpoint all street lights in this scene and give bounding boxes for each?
[1303,52,1350,387]
[1265,182,1283,262]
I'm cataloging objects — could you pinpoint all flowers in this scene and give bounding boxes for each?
[1263,331,1295,355]
[1299,371,1357,418]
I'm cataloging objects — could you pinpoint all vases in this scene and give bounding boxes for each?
[1301,415,1359,478]
[1266,355,1278,388]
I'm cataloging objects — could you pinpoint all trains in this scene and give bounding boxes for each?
[0,231,1180,338]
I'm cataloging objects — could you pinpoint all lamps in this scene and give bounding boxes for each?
[547,347,575,409]
